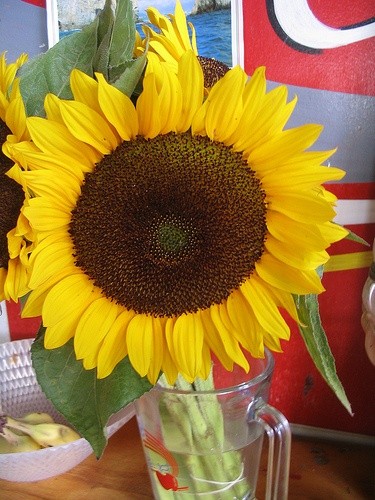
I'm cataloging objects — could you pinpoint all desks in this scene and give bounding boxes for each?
[1,406,374,500]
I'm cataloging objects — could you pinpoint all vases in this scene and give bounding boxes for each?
[132,344,294,500]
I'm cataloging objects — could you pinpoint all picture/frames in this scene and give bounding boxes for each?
[41,0,246,73]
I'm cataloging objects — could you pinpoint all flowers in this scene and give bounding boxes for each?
[1,0,374,500]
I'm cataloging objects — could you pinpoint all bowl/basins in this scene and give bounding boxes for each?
[0,337,139,483]
